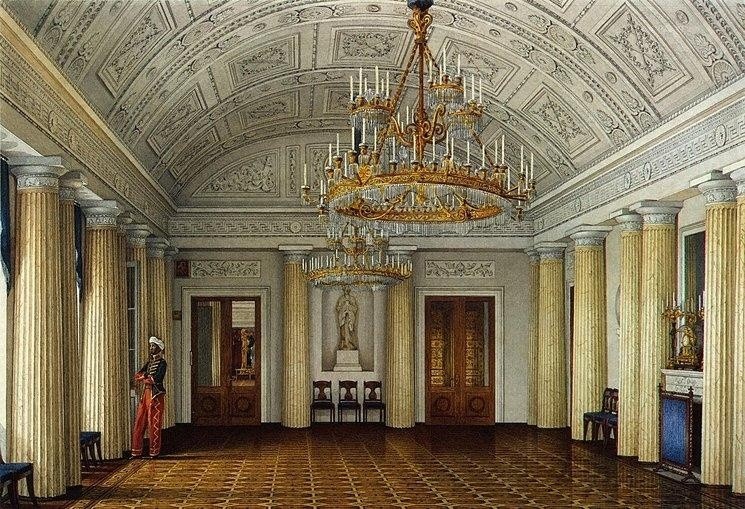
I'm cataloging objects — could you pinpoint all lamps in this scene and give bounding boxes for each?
[302,1,536,236]
[300,94,414,289]
[662,291,704,369]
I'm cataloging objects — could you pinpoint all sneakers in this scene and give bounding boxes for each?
[128,456,142,460]
[149,454,160,460]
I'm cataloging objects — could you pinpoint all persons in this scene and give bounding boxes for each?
[335,288,359,350]
[240,329,249,368]
[129,336,167,460]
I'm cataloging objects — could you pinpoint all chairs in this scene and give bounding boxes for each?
[583,388,619,447]
[0,455,38,508]
[338,380,361,423]
[309,380,335,426]
[363,380,386,424]
[79,431,102,468]
